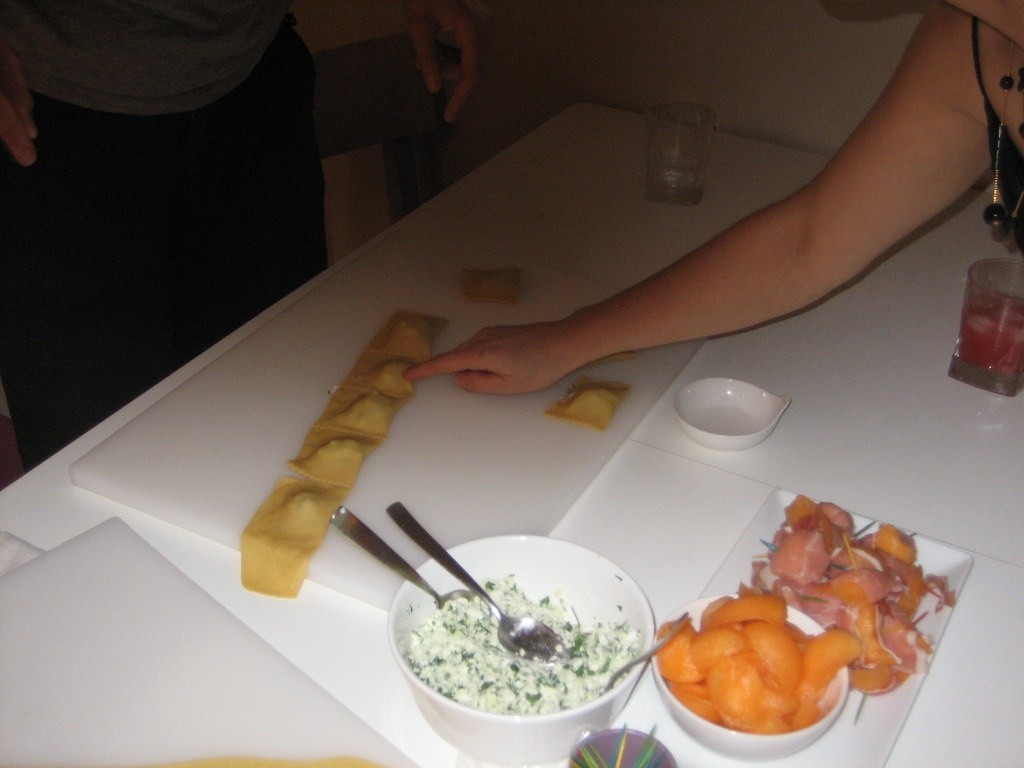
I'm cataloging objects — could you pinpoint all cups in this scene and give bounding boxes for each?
[644,101,714,206]
[570,729,677,768]
[948,258,1024,397]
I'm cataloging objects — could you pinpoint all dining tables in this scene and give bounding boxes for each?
[0,98,1024,767]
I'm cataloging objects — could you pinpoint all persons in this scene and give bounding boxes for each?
[401,0,1024,394]
[0,0,484,474]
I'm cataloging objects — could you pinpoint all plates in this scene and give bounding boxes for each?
[672,377,792,451]
[607,489,973,768]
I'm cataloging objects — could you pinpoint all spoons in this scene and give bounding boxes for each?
[329,506,474,611]
[386,502,572,663]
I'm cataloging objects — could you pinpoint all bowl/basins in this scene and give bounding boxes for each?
[388,534,656,767]
[651,594,851,762]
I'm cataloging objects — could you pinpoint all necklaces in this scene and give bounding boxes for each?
[984,43,1024,230]
